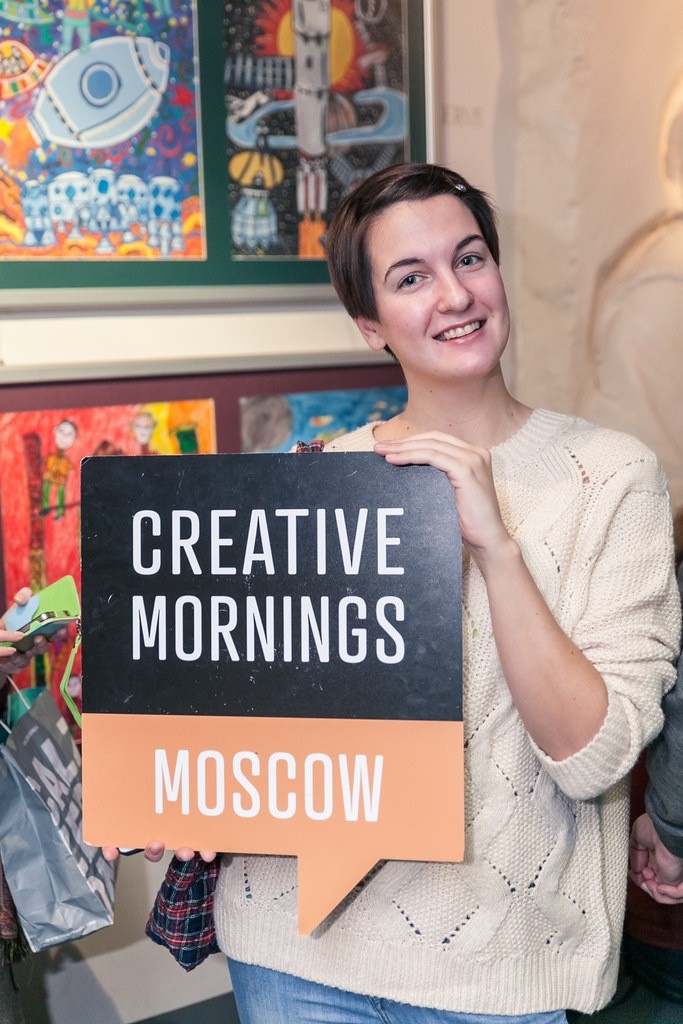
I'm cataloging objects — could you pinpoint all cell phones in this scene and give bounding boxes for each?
[0,575,80,653]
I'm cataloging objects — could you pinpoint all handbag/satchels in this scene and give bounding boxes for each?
[144,849,221,973]
[0,676,120,954]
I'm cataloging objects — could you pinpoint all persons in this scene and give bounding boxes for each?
[626,567,682,903]
[100,165,682,1024]
[0,586,67,689]
